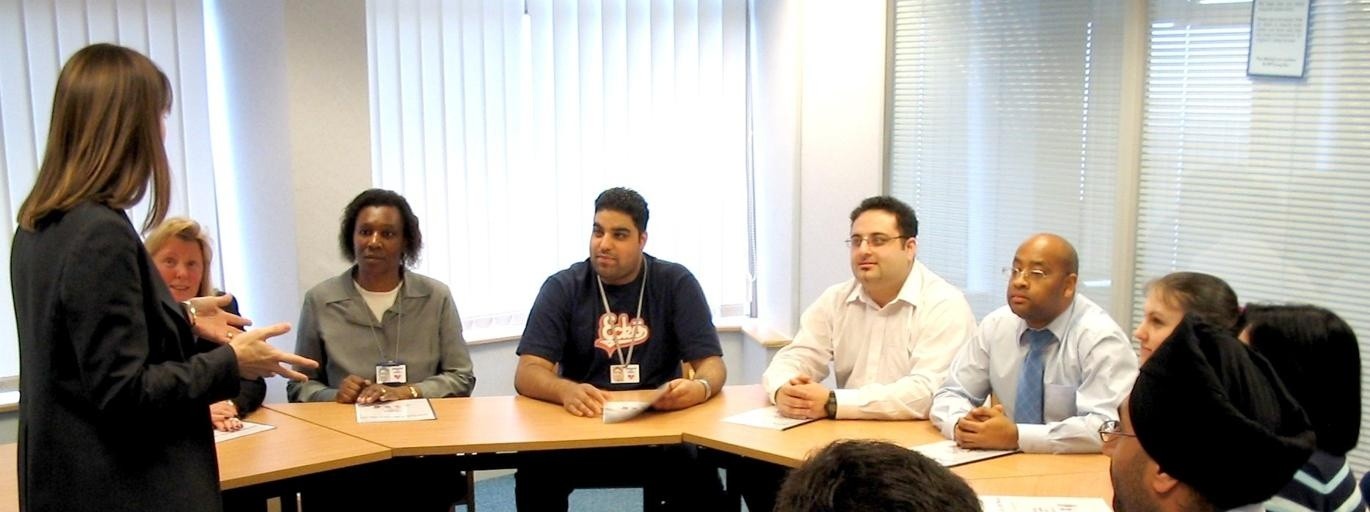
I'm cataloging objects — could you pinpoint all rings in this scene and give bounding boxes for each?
[381,388,387,393]
[227,332,234,338]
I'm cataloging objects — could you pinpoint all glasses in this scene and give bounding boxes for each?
[846,235,899,247]
[1001,267,1061,281]
[1098,419,1137,443]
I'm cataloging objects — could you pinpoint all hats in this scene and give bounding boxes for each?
[1130,314,1315,507]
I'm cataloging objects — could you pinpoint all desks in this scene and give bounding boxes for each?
[682,384,1112,508]
[1,404,392,512]
[263,386,775,510]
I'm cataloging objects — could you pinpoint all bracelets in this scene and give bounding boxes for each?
[180,299,198,328]
[408,385,418,398]
[696,377,712,400]
[226,398,236,406]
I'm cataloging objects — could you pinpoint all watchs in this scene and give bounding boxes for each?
[824,390,837,420]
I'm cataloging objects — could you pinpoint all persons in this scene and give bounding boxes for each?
[141,217,269,511]
[512,187,728,511]
[1234,298,1370,511]
[1094,312,1317,511]
[6,42,326,510]
[768,438,983,511]
[1130,269,1241,368]
[928,231,1143,457]
[739,194,979,511]
[285,187,476,512]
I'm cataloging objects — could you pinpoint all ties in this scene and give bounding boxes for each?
[1014,327,1056,425]
